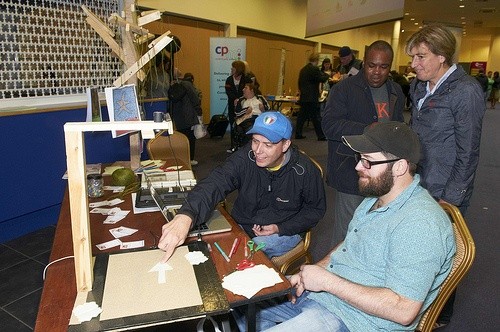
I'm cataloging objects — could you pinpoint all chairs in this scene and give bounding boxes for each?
[270,149,324,277]
[146,129,192,170]
[414,202,475,332]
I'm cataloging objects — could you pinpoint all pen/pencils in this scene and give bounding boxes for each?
[228,237,238,258]
[244,236,248,257]
[233,239,241,254]
[214,241,230,263]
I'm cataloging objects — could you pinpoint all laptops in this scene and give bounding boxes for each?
[144,172,233,239]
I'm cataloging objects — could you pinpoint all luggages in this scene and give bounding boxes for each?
[207,104,230,139]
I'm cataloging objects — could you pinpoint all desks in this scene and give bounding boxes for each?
[33,158,294,332]
[266,98,301,132]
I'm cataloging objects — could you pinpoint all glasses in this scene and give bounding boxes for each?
[354,155,404,169]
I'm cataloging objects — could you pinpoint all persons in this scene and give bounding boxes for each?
[224,45,500,154]
[406,24,486,332]
[157,112,326,260]
[322,41,404,245]
[169,73,202,166]
[234,122,456,332]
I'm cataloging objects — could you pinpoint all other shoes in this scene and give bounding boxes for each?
[318,137,328,141]
[190,160,198,166]
[432,321,447,330]
[227,149,232,153]
[295,135,306,139]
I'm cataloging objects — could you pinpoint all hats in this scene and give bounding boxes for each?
[340,120,421,164]
[246,111,292,143]
[338,46,352,58]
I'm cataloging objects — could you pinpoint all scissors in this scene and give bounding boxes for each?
[246,241,266,260]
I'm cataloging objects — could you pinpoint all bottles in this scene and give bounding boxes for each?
[87,174,105,198]
